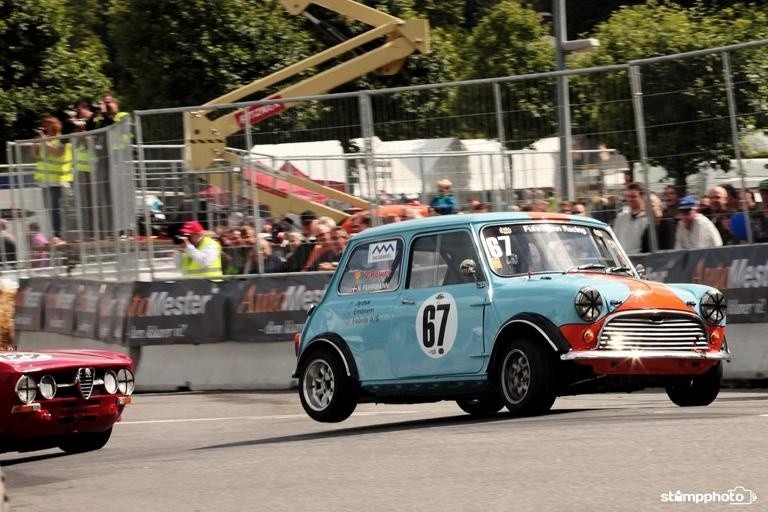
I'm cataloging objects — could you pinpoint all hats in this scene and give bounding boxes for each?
[678,198,699,211]
[181,220,202,234]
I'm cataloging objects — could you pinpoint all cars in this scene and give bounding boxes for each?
[289,209,730,424]
[1,340,136,453]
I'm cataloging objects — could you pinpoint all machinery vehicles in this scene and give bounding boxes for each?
[181,0,431,233]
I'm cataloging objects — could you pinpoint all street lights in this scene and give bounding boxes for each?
[553,38,603,200]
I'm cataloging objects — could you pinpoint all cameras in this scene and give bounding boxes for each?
[173,234,191,245]
[32,127,48,137]
[90,104,109,113]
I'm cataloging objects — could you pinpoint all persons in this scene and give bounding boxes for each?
[170,179,768,284]
[0,94,135,270]
